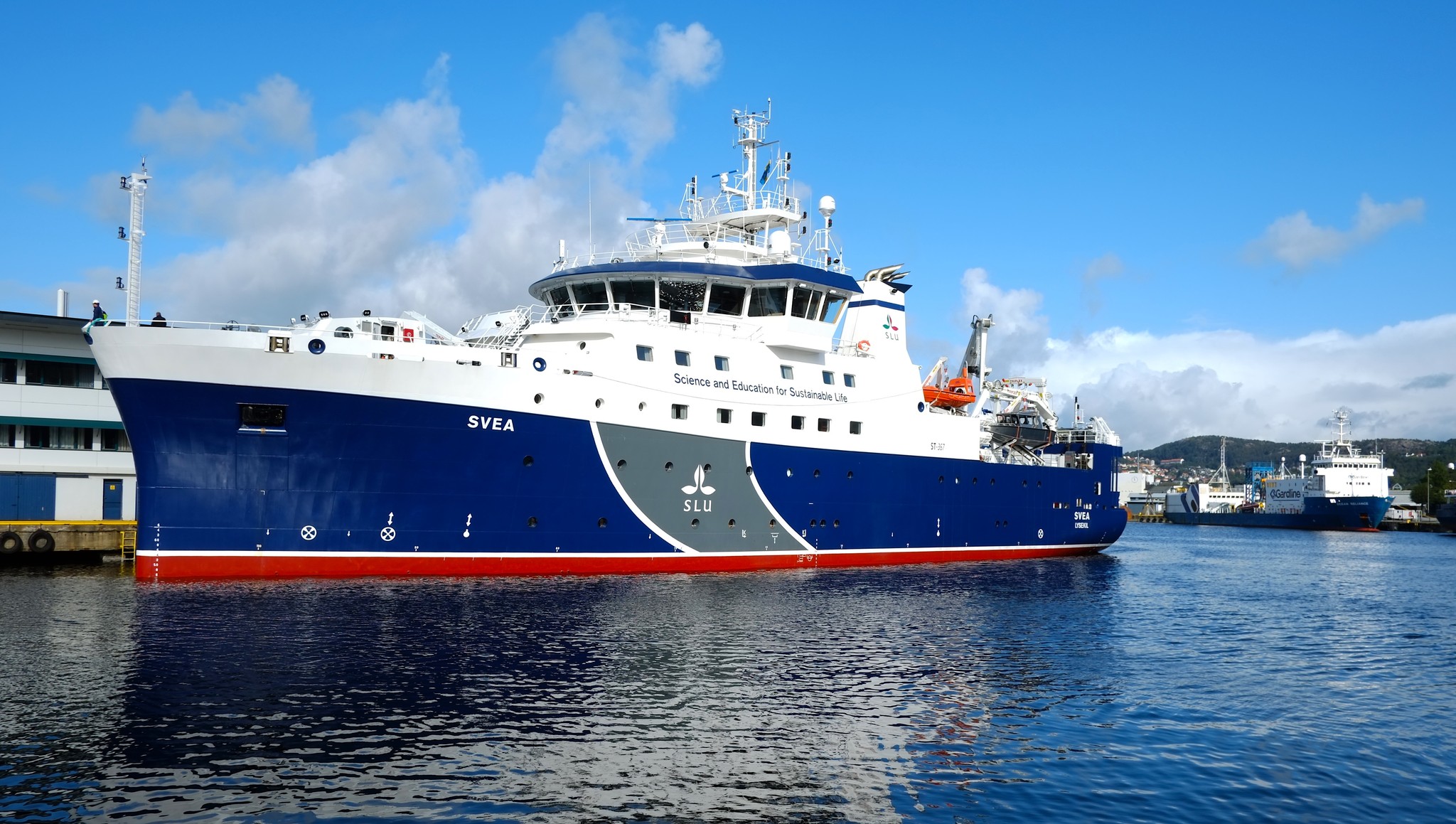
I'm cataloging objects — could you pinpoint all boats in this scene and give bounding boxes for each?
[920,360,976,410]
[82,97,1134,589]
[1,309,138,569]
[1122,408,1441,533]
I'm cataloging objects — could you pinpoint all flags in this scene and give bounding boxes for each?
[759,159,771,185]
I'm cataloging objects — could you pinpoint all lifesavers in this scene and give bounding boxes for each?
[1146,518,1150,522]
[28,529,54,554]
[1165,519,1170,523]
[858,340,870,351]
[0,531,22,556]
[1158,518,1163,522]
[1152,518,1157,523]
[1140,518,1144,522]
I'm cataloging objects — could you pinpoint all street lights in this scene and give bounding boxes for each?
[1427,467,1433,516]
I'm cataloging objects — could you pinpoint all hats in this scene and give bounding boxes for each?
[91,300,99,303]
[100,306,103,310]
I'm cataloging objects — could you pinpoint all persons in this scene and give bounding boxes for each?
[89,300,104,326]
[150,312,167,327]
